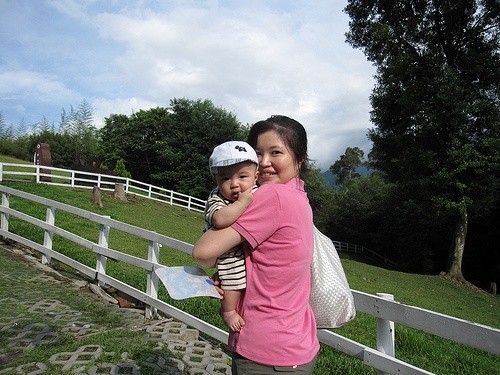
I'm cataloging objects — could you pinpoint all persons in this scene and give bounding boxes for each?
[204,161,259,332]
[192,115,321,375]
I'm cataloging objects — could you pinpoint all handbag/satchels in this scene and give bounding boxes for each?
[308,224,357,329]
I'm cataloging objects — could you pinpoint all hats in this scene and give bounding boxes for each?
[208,141,260,177]
